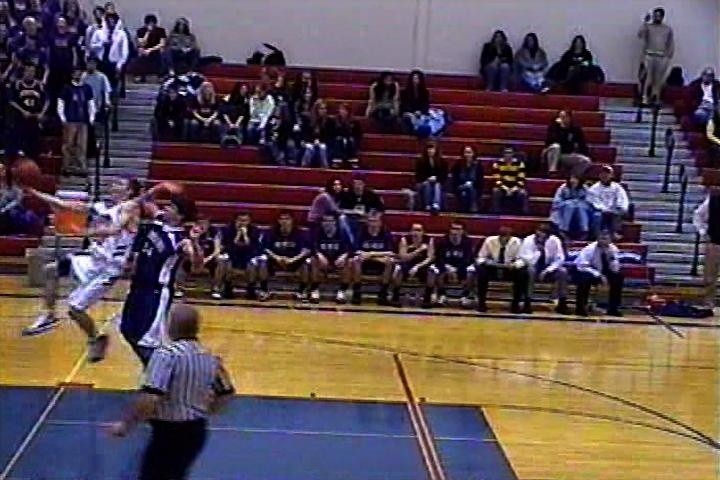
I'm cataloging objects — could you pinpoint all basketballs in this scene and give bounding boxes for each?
[12,158,43,188]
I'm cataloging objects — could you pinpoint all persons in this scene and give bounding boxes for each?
[114,180,199,371]
[2,1,628,316]
[665,65,719,167]
[693,188,719,309]
[105,303,235,480]
[635,7,674,108]
[18,176,140,362]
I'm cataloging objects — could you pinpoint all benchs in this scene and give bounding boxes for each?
[0,61,720,302]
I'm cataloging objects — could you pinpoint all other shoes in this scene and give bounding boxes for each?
[177,281,360,304]
[375,294,623,319]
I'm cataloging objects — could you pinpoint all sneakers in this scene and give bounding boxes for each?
[23,311,61,335]
[88,331,108,361]
[330,157,625,242]
